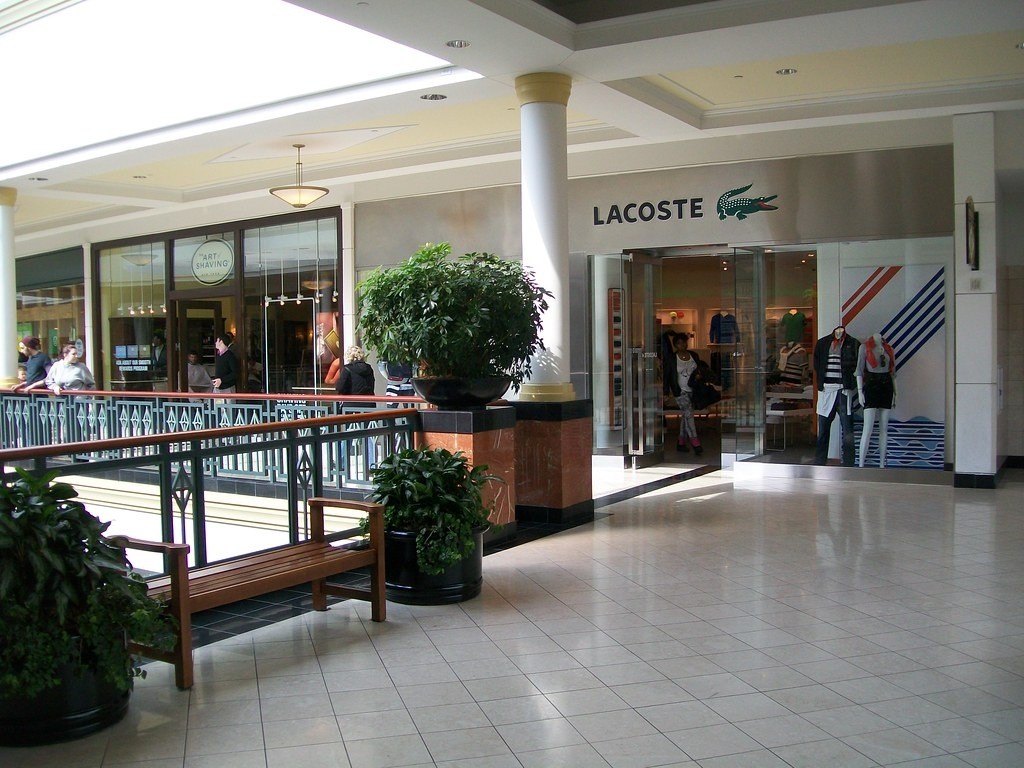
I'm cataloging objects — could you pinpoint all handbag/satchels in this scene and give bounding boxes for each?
[692,384,721,410]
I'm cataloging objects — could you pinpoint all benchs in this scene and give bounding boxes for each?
[760,407,814,451]
[102,497,388,688]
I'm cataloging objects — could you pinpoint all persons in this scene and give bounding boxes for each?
[316,312,340,384]
[45,345,96,442]
[152,334,167,391]
[812,326,861,466]
[385,380,414,408]
[18,366,27,383]
[247,355,263,386]
[188,352,211,385]
[333,346,375,470]
[709,311,740,343]
[778,340,809,385]
[11,336,52,445]
[664,332,702,446]
[853,333,897,468]
[781,309,807,342]
[211,332,239,445]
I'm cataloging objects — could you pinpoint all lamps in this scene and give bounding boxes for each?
[269,144,330,209]
[120,245,157,266]
[300,260,333,291]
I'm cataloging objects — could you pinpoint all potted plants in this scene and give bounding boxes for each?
[356,444,514,606]
[0,467,186,745]
[349,241,556,410]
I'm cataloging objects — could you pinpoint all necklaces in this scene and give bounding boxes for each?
[683,357,686,358]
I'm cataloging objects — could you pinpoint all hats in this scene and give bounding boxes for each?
[21,336,40,348]
[677,311,684,317]
[669,312,677,318]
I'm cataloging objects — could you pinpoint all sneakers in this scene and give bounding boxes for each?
[676,437,690,453]
[689,438,704,454]
